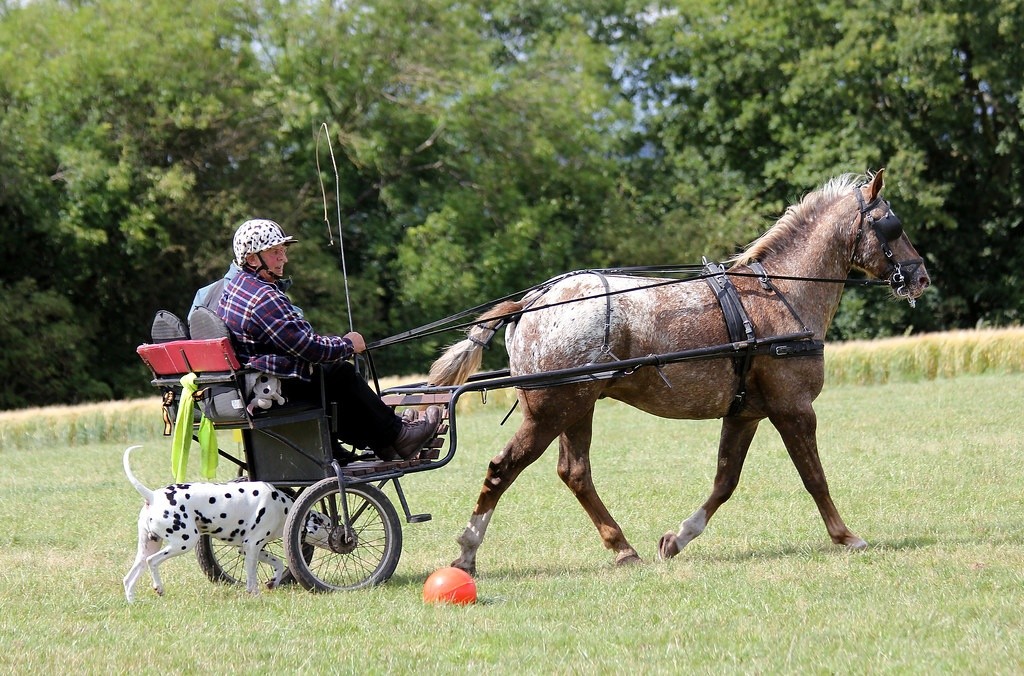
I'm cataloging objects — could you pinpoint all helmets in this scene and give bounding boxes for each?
[272,221,298,246]
[233,219,293,266]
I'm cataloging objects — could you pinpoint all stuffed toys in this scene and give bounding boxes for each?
[248,373,285,415]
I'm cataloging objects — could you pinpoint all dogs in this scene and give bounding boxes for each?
[114,440,341,614]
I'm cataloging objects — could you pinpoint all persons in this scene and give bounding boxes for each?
[215,219,443,463]
[188,220,370,465]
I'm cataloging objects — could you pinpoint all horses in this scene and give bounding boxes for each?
[406,165,934,578]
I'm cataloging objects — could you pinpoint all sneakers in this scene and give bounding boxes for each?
[374,408,418,461]
[391,405,442,460]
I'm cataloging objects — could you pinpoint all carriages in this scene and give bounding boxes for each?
[135,169,931,595]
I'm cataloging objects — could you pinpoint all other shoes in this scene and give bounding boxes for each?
[333,444,358,463]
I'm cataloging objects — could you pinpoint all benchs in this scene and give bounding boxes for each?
[137,305,325,428]
